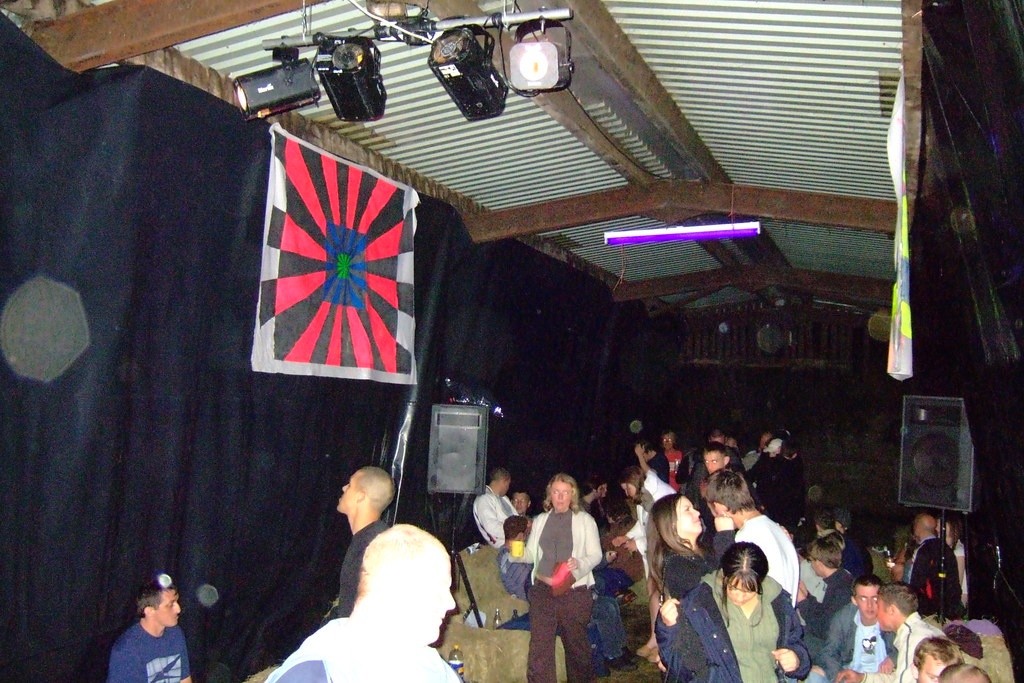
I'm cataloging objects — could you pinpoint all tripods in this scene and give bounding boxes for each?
[448,495,484,630]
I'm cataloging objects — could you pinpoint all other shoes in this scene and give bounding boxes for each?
[593,660,610,678]
[647,647,660,662]
[605,656,638,671]
[636,643,657,657]
[621,645,635,660]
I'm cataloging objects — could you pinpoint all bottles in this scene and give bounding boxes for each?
[448,643,464,682]
[512,610,518,619]
[493,608,502,629]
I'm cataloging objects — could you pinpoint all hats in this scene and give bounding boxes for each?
[763,437,783,453]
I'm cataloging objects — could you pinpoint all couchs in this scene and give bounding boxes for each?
[426,542,567,683]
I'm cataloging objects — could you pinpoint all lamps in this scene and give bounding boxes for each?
[317,35,387,122]
[428,24,509,123]
[509,18,575,91]
[603,221,761,245]
[232,46,321,121]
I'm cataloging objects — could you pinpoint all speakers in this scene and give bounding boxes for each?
[428,405,488,494]
[898,395,980,514]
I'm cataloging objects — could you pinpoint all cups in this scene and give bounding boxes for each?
[511,540,524,557]
[883,550,894,572]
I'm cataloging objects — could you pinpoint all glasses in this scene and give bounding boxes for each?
[663,438,674,442]
[806,555,817,563]
[854,595,878,603]
[148,571,178,590]
[704,460,719,465]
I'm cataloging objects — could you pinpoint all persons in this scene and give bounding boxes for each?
[508,472,603,683]
[913,636,965,682]
[511,488,531,518]
[264,522,462,683]
[473,468,520,549]
[939,665,992,683]
[108,576,193,682]
[497,515,534,602]
[579,423,968,624]
[833,582,946,682]
[654,541,811,683]
[336,466,396,618]
[811,574,899,683]
